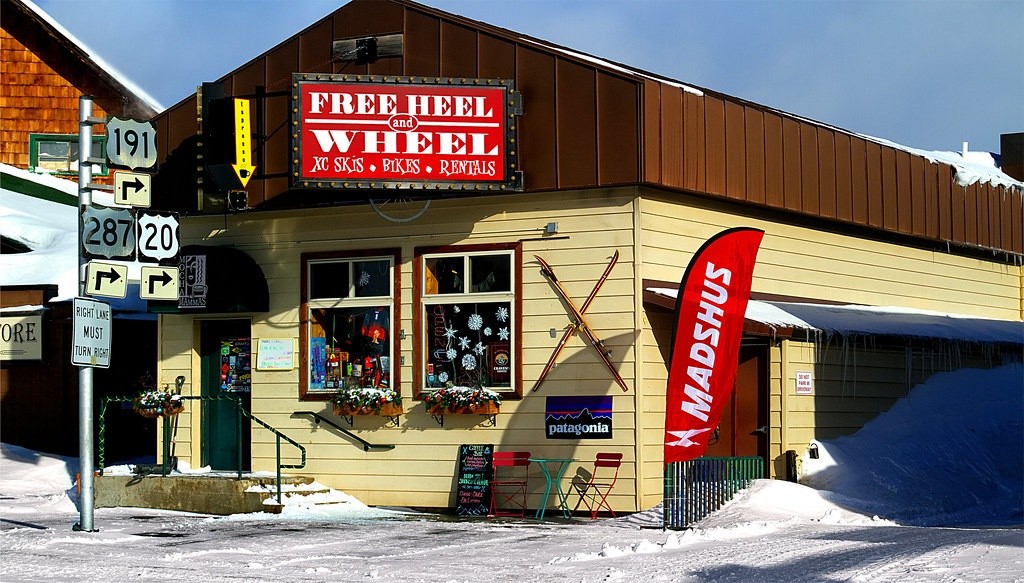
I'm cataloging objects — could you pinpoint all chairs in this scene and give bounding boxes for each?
[489,452,531,521]
[568,453,623,520]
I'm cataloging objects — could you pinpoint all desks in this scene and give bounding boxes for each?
[528,457,574,521]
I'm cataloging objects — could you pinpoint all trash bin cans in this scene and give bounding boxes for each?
[456,443,494,517]
